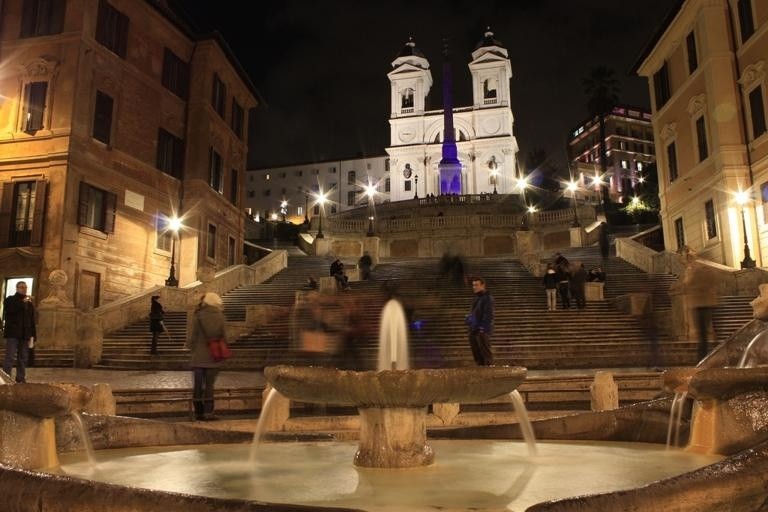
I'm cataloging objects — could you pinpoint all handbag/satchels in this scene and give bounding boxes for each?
[208,341,231,360]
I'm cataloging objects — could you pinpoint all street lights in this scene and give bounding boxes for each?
[414,174,418,199]
[314,191,328,240]
[161,211,186,287]
[590,167,604,223]
[563,171,582,229]
[362,184,380,238]
[489,165,501,195]
[516,174,537,229]
[278,198,290,223]
[731,186,758,269]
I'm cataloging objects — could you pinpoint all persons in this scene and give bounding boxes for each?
[634,278,668,372]
[300,251,494,371]
[184,293,229,420]
[151,296,164,355]
[683,250,727,361]
[4,281,37,383]
[599,222,610,258]
[543,253,607,310]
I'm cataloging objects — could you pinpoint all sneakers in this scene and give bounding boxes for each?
[194,414,218,421]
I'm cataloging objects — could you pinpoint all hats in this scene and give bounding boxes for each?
[203,293,221,306]
